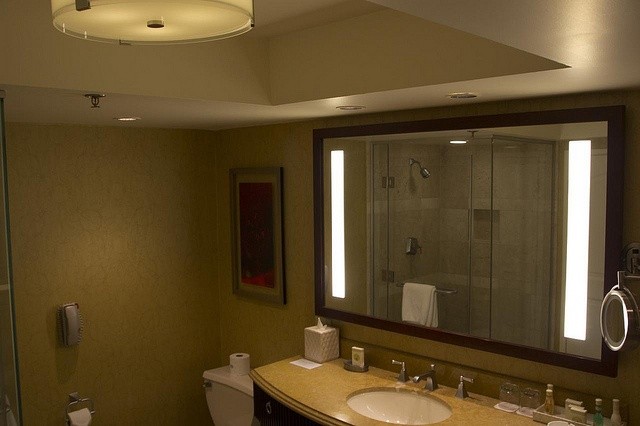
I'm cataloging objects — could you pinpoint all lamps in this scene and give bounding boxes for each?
[50,0,256,49]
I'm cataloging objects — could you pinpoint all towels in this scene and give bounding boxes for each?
[401,283,438,329]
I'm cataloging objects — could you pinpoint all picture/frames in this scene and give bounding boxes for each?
[228,167,285,306]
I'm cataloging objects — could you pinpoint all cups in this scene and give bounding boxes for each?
[520,387,540,416]
[547,420,576,426]
[498,382,520,410]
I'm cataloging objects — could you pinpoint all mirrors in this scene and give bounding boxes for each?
[314,106,629,379]
[600,270,640,355]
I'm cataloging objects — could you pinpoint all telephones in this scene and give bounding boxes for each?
[57,302,80,346]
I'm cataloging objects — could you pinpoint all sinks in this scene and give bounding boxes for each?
[346,386,453,426]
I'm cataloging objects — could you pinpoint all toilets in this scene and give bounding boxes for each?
[204,365,254,426]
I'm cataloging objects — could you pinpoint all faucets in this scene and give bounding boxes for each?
[413,364,437,390]
